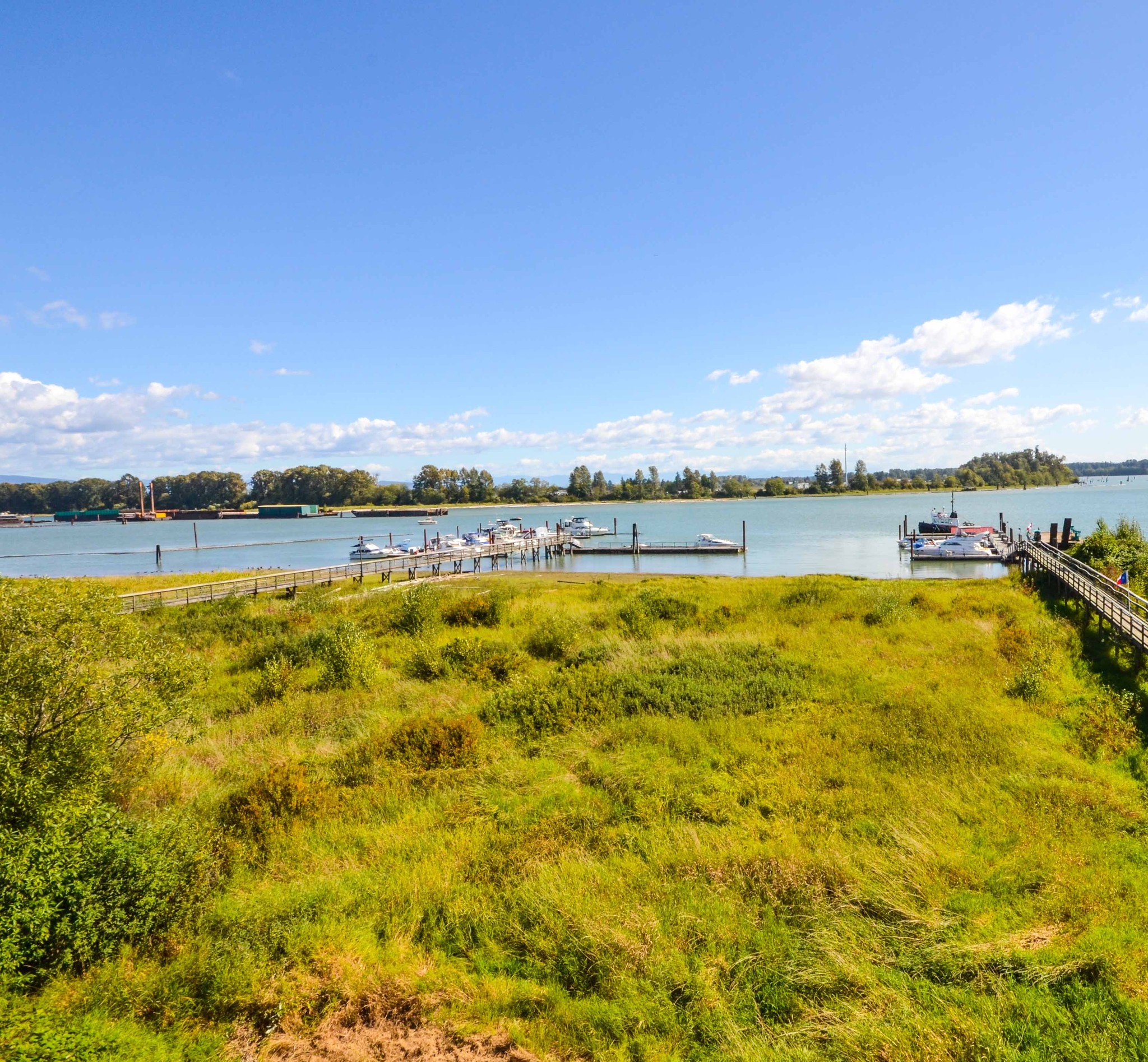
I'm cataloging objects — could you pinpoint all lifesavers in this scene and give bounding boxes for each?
[938,514,942,519]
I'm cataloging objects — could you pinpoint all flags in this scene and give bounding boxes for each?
[1116,570,1128,584]
[1026,523,1032,531]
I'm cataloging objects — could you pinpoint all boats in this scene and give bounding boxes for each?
[346,542,389,558]
[563,517,609,537]
[383,518,540,558]
[931,491,960,526]
[696,533,738,547]
[903,531,993,557]
[626,543,649,547]
[418,518,438,525]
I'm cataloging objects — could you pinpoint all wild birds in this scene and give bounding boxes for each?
[247,565,290,570]
[136,569,213,574]
[20,574,86,578]
